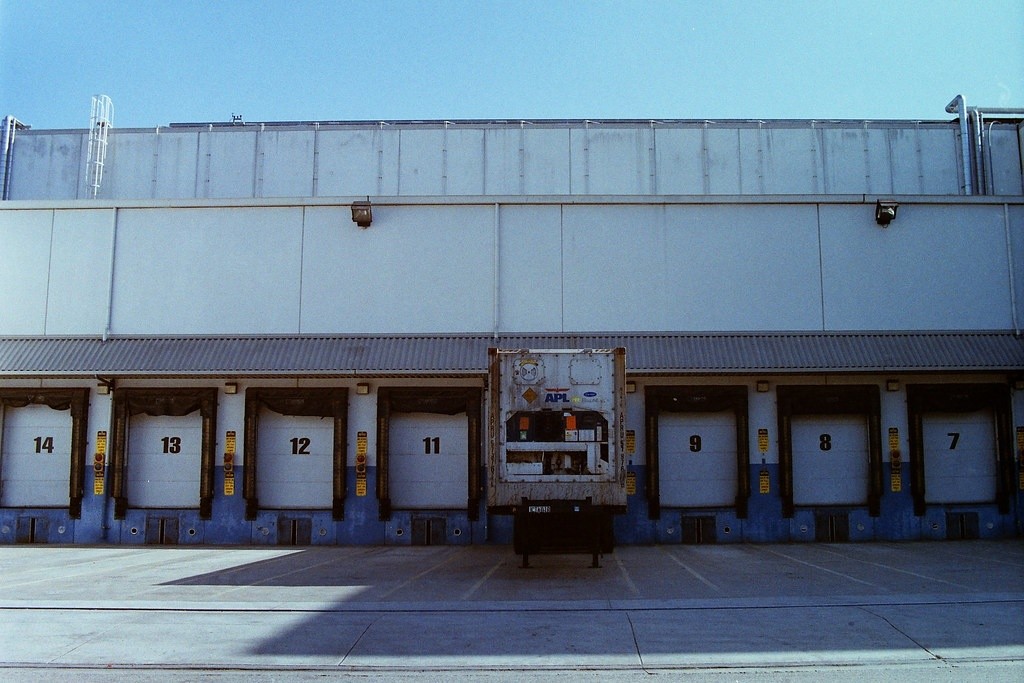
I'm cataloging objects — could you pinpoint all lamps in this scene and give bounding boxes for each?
[351,196,372,229]
[875,199,899,229]
[223,381,238,394]
[757,380,769,392]
[626,381,636,393]
[97,383,111,395]
[356,383,370,395]
[886,379,901,391]
